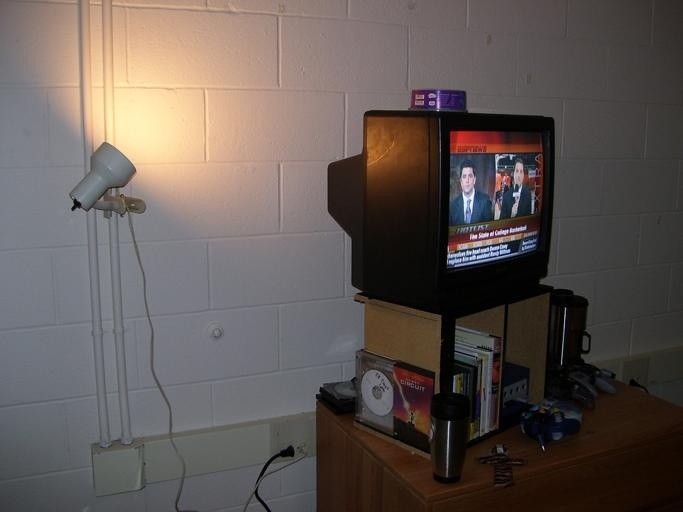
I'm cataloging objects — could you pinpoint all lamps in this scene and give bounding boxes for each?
[69,142,146,218]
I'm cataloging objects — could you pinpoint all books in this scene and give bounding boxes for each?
[450,324,503,442]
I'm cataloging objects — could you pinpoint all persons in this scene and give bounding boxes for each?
[493,157,539,220]
[447,160,492,224]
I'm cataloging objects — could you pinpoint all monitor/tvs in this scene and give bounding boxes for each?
[327,110,556,314]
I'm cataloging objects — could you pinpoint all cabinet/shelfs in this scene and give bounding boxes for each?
[353,284,552,449]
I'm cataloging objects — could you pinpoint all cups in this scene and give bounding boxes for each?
[429,391,471,483]
[548,288,591,368]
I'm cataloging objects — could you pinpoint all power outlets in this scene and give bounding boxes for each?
[270,416,314,464]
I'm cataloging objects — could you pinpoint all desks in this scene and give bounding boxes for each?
[317,380,683,512]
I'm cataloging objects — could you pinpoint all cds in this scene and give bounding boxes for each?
[360,368,394,416]
[335,380,360,397]
